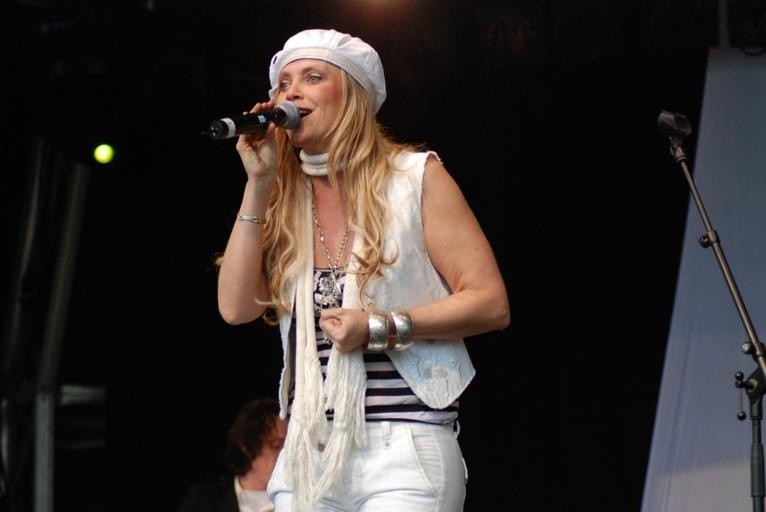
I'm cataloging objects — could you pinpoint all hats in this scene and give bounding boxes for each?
[267,28,387,115]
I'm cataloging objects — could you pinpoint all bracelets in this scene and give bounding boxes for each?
[391,311,414,351]
[367,311,389,352]
[237,212,267,225]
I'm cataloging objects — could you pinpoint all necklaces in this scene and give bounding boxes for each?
[309,182,353,307]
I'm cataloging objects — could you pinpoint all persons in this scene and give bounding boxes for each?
[177,396,293,511]
[218,27,511,511]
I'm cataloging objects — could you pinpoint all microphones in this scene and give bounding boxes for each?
[208,100,300,140]
